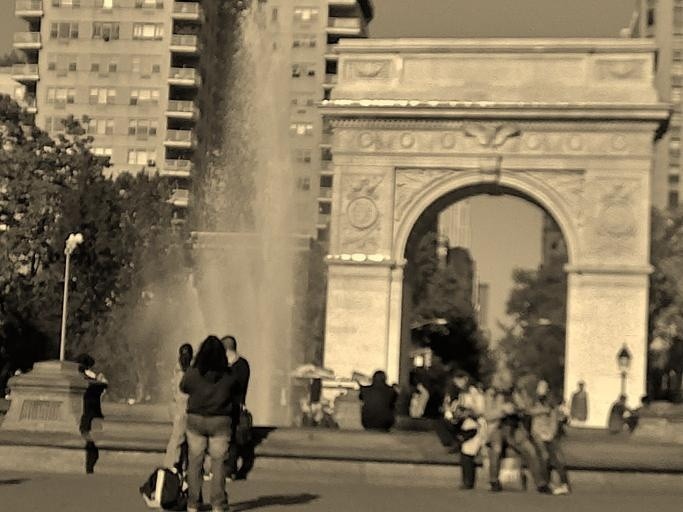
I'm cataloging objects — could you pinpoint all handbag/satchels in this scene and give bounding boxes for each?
[235,410,254,448]
[139,466,202,511]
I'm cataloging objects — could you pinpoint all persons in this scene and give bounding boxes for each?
[354,371,397,434]
[569,381,589,423]
[73,353,94,375]
[160,342,204,470]
[433,367,576,496]
[215,334,250,482]
[172,333,236,511]
[605,393,634,434]
[297,399,341,430]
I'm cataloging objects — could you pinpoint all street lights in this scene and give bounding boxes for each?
[615,343,632,398]
[59,232,85,362]
